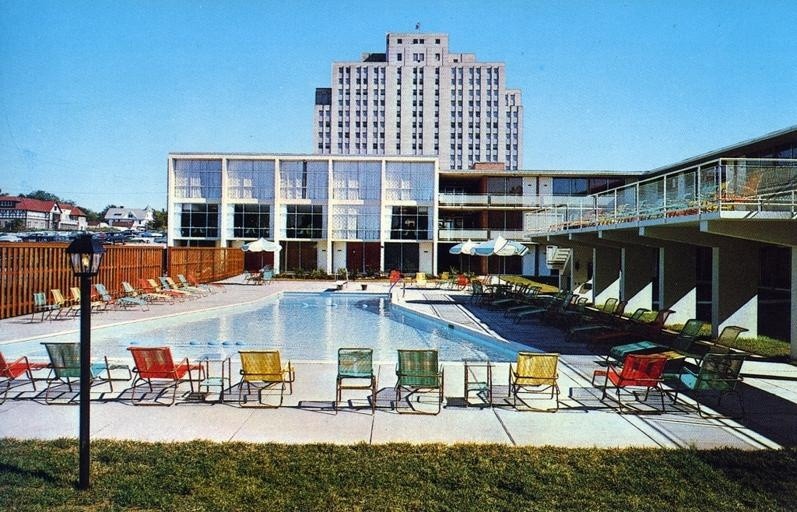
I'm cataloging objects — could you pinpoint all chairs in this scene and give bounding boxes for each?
[31,274,226,322]
[246,271,273,286]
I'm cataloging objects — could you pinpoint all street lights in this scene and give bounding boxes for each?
[60,227,110,488]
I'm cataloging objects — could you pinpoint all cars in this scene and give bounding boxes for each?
[0,228,167,245]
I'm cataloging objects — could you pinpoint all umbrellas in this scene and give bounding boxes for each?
[468,232,530,284]
[239,236,283,269]
[448,238,480,274]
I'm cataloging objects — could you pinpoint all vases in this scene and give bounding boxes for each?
[361,285,367,290]
